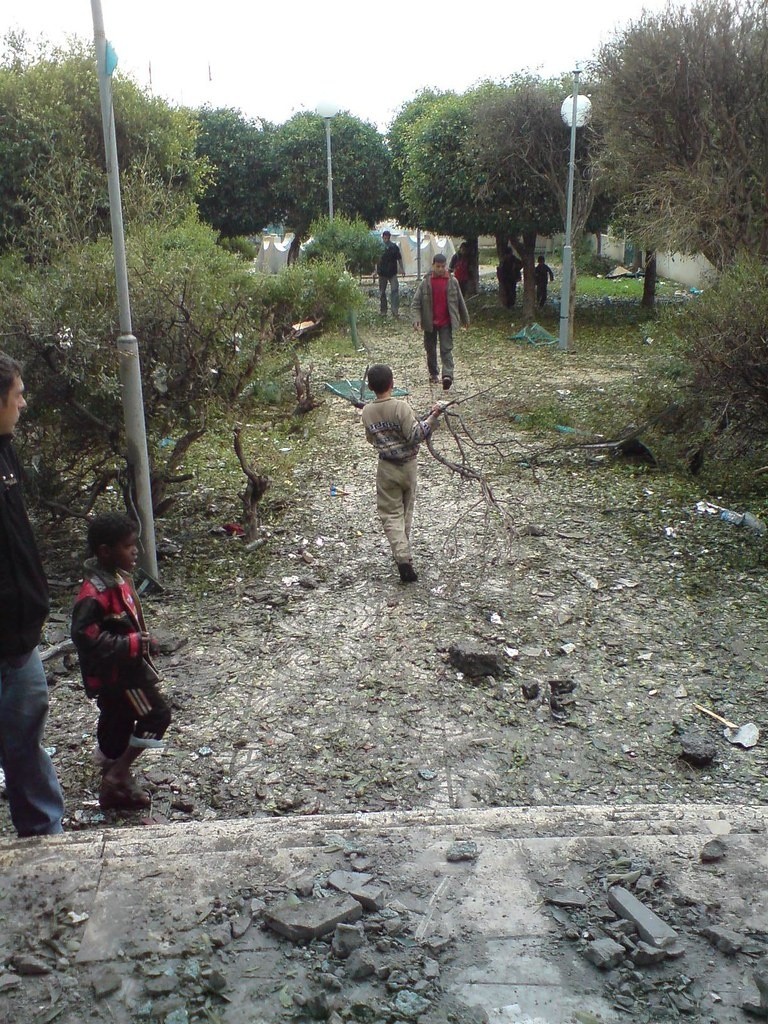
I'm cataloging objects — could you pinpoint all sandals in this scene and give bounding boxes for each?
[103,777,151,805]
[98,795,147,810]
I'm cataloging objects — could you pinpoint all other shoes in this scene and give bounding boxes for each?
[380,312,387,316]
[442,377,453,390]
[392,313,400,317]
[398,562,418,584]
[429,374,442,382]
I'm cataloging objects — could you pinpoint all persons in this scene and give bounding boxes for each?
[410,253,470,390]
[448,242,475,298]
[362,364,441,581]
[372,231,406,319]
[533,256,554,309]
[70,514,170,808]
[0,352,69,838]
[500,247,523,308]
